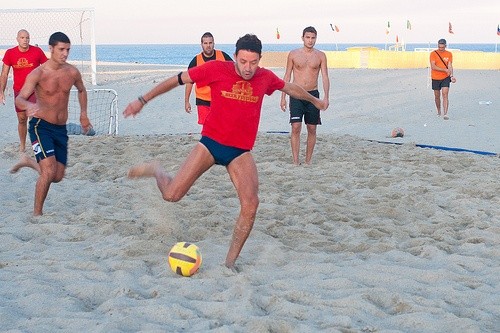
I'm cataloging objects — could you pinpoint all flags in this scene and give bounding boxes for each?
[276,19,500,43]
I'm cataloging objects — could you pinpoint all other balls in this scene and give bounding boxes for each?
[392,125,405,139]
[167,241,205,278]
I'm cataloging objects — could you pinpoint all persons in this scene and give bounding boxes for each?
[185,32,235,125]
[280,26,330,165]
[0,29,48,151]
[121,33,324,268]
[429,39,456,115]
[10,32,92,217]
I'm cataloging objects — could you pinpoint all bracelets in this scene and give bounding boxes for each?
[450,76,453,77]
[138,95,146,104]
